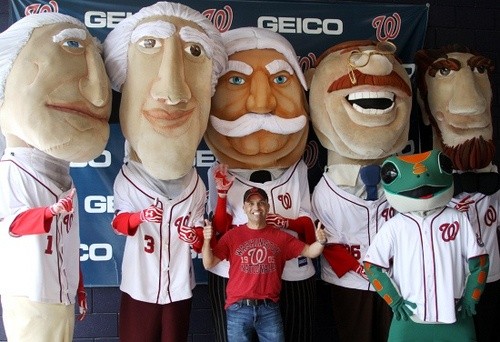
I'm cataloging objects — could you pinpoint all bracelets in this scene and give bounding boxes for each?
[320,242,326,245]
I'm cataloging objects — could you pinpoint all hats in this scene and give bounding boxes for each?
[243,187,268,204]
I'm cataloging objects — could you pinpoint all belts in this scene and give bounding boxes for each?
[236,298,270,306]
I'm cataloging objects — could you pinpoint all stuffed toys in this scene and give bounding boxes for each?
[362,150,490,342]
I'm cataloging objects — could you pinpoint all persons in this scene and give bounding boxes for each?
[204,26,317,342]
[101,2,229,342]
[203,187,327,342]
[305,40,413,342]
[418,42,500,342]
[0,2,113,342]
